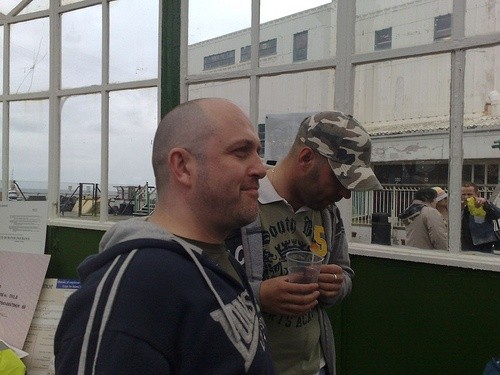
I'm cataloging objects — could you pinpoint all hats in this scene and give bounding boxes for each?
[297,111,384,191]
[432,187,448,208]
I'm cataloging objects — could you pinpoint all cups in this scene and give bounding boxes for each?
[286,250,324,309]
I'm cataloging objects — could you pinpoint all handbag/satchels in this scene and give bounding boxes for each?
[493,218,500,250]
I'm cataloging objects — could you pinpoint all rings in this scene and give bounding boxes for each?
[333,273,338,282]
[478,201,480,203]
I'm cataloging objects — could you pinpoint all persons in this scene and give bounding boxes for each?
[227,110,383,374]
[401,183,500,252]
[54,98,267,374]
